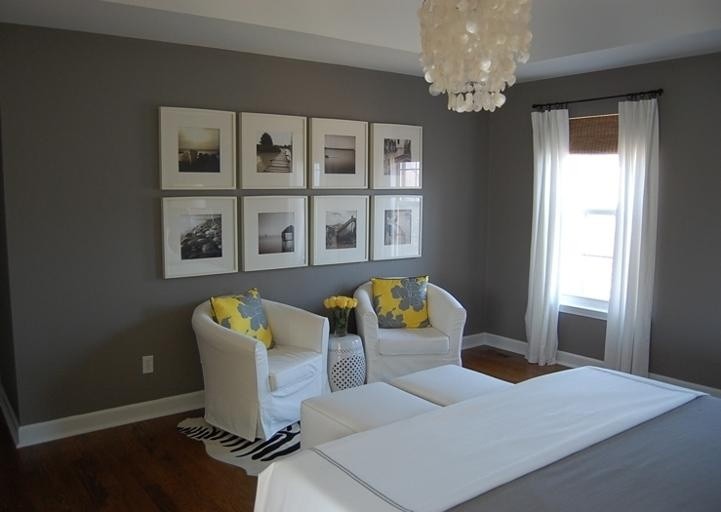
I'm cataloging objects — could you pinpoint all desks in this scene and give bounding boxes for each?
[327,333,365,394]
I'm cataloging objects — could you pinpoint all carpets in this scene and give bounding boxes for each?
[176,414,302,479]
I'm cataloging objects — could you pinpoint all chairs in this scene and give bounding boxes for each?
[353,277,470,385]
[188,294,334,444]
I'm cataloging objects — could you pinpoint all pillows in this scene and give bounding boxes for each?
[204,288,276,351]
[372,275,433,331]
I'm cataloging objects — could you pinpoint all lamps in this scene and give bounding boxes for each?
[417,0,538,117]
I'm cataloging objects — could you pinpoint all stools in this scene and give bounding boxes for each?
[390,361,517,409]
[299,382,446,453]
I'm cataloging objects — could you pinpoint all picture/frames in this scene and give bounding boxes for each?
[241,194,310,273]
[239,110,308,192]
[160,195,239,281]
[158,104,238,192]
[309,116,370,190]
[308,193,370,266]
[370,120,425,192]
[370,194,425,263]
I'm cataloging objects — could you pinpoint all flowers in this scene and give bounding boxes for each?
[323,293,360,334]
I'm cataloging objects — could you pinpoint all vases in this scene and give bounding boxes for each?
[330,310,349,334]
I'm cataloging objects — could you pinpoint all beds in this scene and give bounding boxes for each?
[252,363,721,511]
